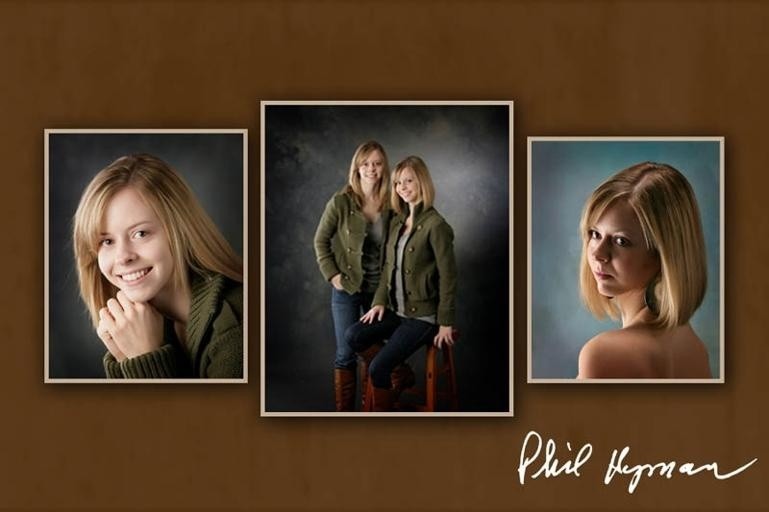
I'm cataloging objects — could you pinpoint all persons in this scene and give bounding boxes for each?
[73,155,243,378]
[573,162,713,377]
[313,140,397,412]
[341,157,459,412]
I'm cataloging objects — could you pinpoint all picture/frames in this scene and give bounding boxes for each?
[259,101,516,419]
[41,128,248,386]
[527,137,726,384]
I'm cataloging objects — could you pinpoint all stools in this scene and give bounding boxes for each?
[363,329,462,412]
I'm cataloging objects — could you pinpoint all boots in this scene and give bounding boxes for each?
[335,342,416,411]
